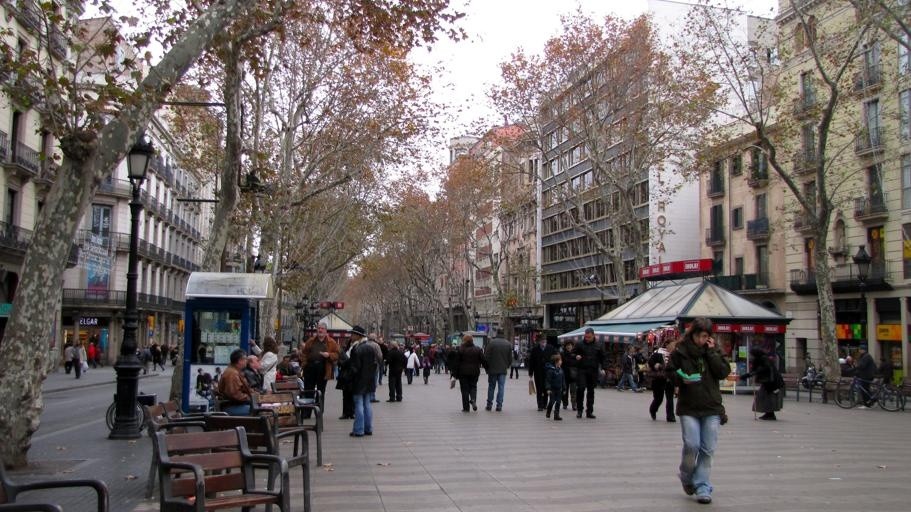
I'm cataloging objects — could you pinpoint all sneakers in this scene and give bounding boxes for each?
[678,466,696,496]
[697,495,712,504]
[858,401,880,411]
[336,397,402,438]
[760,413,777,420]
[459,401,598,421]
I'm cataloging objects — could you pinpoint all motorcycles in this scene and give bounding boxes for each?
[800,355,821,388]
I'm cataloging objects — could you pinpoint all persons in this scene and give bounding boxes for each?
[63,340,104,380]
[616,340,679,423]
[857,345,879,409]
[879,358,893,384]
[739,344,785,420]
[135,340,179,375]
[665,316,732,503]
[196,367,222,401]
[843,356,855,369]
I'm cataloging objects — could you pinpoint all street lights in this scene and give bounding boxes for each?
[294,292,318,344]
[522,304,533,349]
[426,318,430,335]
[107,128,157,443]
[253,250,268,347]
[475,311,481,331]
[850,242,873,356]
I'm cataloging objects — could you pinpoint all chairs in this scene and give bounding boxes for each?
[142,376,322,512]
[882,377,911,410]
[782,376,800,402]
[0,457,109,512]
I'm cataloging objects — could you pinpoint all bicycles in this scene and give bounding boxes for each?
[830,374,908,413]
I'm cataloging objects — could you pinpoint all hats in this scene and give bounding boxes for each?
[346,325,367,338]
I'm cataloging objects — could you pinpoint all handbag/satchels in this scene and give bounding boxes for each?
[528,379,536,397]
[718,406,730,426]
